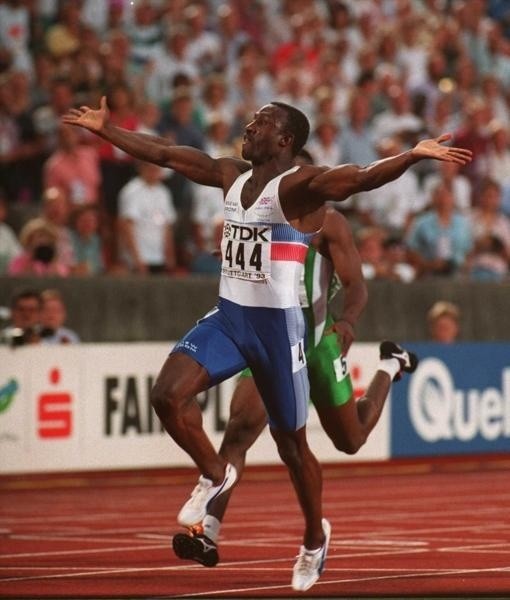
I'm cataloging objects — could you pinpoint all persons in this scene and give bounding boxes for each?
[0,290,52,346]
[167,150,420,572]
[2,1,509,277]
[39,287,81,343]
[426,299,462,343]
[57,96,476,591]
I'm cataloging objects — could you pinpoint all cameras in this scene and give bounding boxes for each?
[35,245,54,262]
[8,328,30,348]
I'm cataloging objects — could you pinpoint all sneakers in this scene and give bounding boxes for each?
[380,340,419,382]
[292,517,332,591]
[172,534,219,567]
[177,462,237,527]
